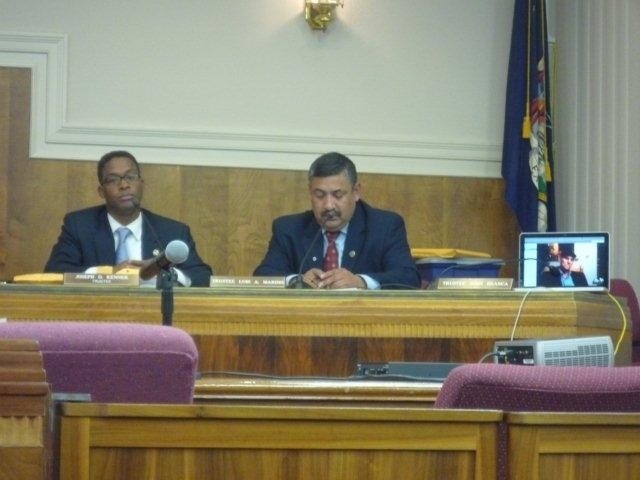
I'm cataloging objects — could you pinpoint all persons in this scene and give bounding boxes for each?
[253,153,421,289]
[44,152,213,287]
[540,250,587,287]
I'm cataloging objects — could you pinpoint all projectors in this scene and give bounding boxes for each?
[493,334,615,368]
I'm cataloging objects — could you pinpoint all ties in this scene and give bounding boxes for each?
[324,231,340,270]
[114,227,131,263]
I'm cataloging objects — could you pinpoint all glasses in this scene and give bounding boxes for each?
[101,170,141,185]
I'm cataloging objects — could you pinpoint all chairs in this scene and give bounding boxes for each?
[430,362,640,478]
[0,321,197,421]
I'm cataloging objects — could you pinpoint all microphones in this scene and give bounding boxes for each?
[295,211,335,289]
[138,239,189,281]
[132,197,185,288]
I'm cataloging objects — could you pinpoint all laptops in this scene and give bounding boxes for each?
[514,231,611,293]
